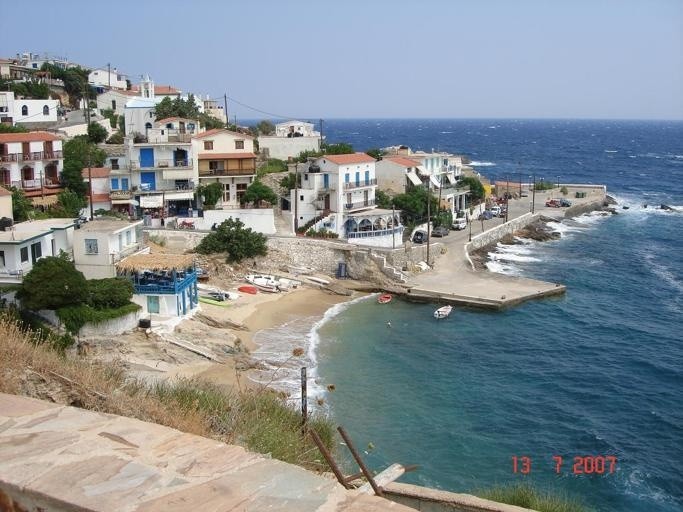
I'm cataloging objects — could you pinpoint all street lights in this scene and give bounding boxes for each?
[539,178,544,190]
[503,175,511,222]
[528,173,535,215]
[556,175,560,188]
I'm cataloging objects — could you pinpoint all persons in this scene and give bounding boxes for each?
[144,208,164,227]
[61,113,68,122]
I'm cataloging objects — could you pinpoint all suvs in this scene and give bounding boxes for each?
[545,199,560,208]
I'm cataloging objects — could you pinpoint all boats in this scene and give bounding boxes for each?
[245,273,289,293]
[279,277,300,290]
[190,257,210,280]
[433,305,452,320]
[376,294,391,304]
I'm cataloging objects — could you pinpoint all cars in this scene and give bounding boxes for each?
[411,190,527,244]
[558,196,570,207]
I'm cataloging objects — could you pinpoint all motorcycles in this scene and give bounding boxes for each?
[178,221,195,229]
